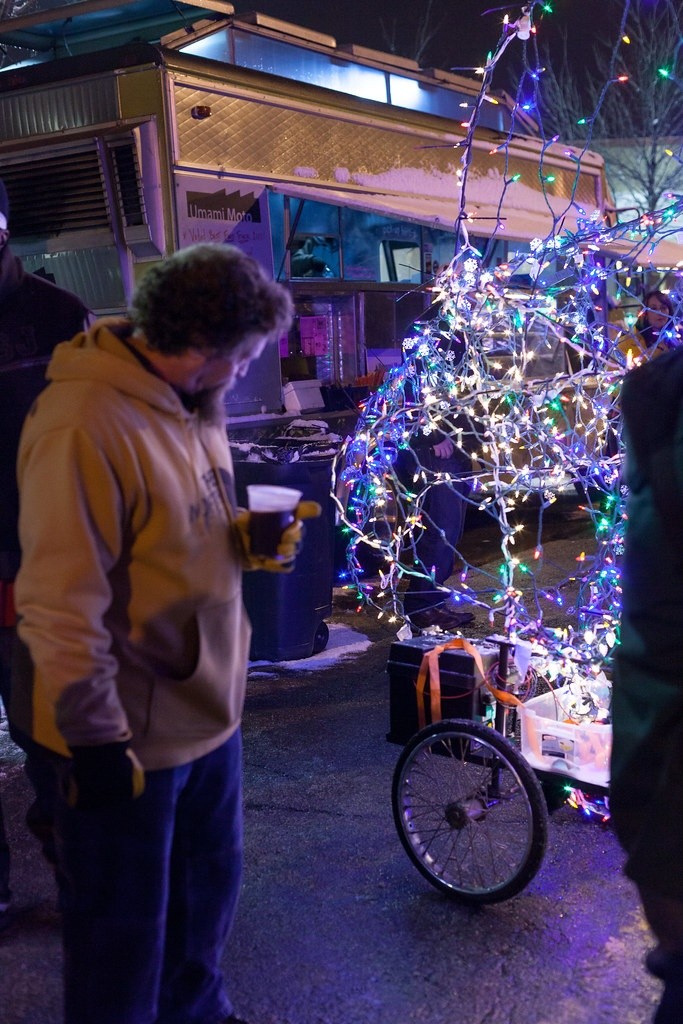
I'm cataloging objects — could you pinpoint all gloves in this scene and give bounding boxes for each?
[234,500,321,572]
[66,739,145,811]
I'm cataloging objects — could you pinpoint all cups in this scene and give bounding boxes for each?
[246,484,303,560]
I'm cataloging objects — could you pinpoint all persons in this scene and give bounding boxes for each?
[399,266,480,629]
[0,209,335,1023]
[618,290,681,366]
[606,340,683,1023]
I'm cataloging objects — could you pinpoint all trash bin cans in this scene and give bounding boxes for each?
[229,436,345,662]
